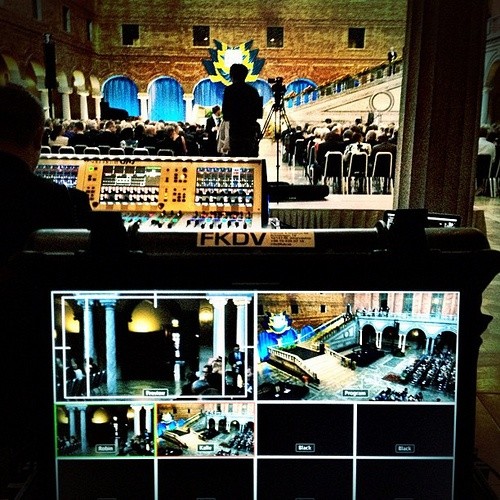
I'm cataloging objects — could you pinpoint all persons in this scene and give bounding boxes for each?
[342,302,456,402]
[474,127,496,195]
[0,81,106,254]
[41,45,398,192]
[55,344,253,457]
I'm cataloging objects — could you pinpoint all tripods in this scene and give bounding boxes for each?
[259,94,313,187]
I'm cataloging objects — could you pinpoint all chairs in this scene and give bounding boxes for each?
[56,370,106,400]
[40,144,172,155]
[291,138,392,196]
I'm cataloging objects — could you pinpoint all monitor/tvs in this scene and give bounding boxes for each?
[0,246,480,500]
